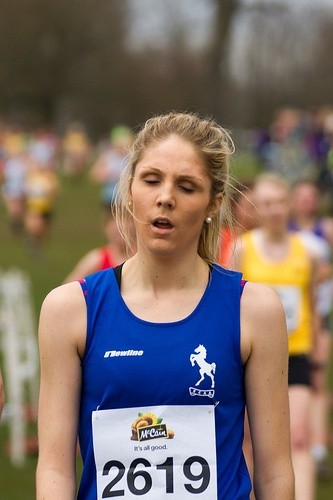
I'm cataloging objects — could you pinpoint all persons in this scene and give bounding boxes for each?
[37,114,295,500]
[0,107,333,499]
